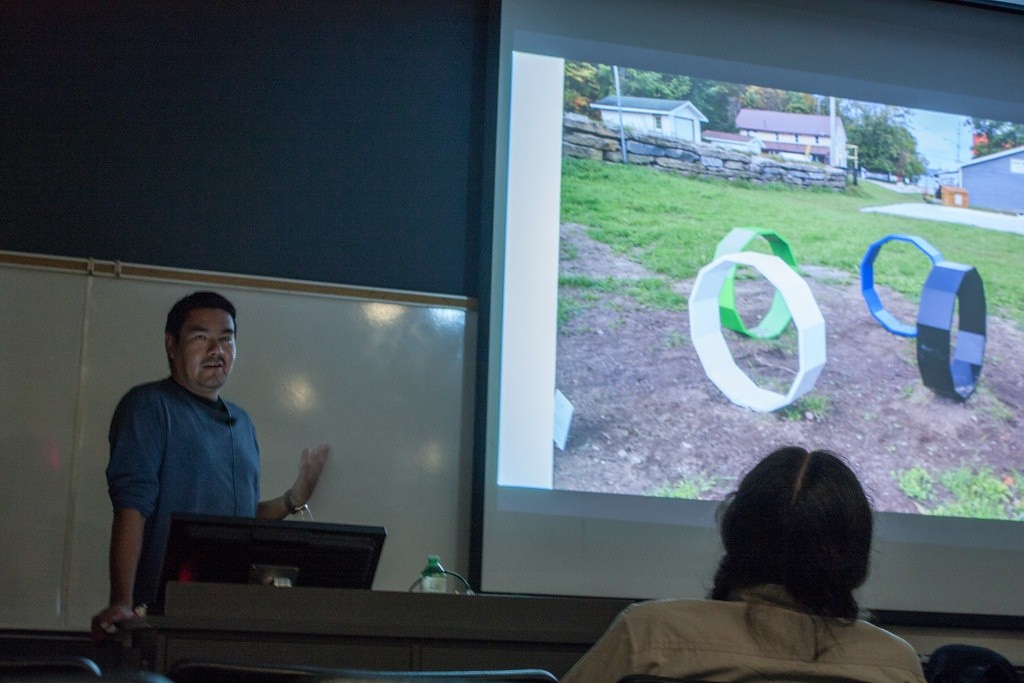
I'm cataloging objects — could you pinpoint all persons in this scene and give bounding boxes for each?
[559,446,927,683]
[90,289,330,636]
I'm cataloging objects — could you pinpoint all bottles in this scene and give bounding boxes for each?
[420,554,446,593]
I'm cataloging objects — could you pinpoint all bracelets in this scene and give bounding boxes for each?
[284,489,308,515]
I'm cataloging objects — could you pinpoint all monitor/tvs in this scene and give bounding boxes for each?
[152,510,386,616]
